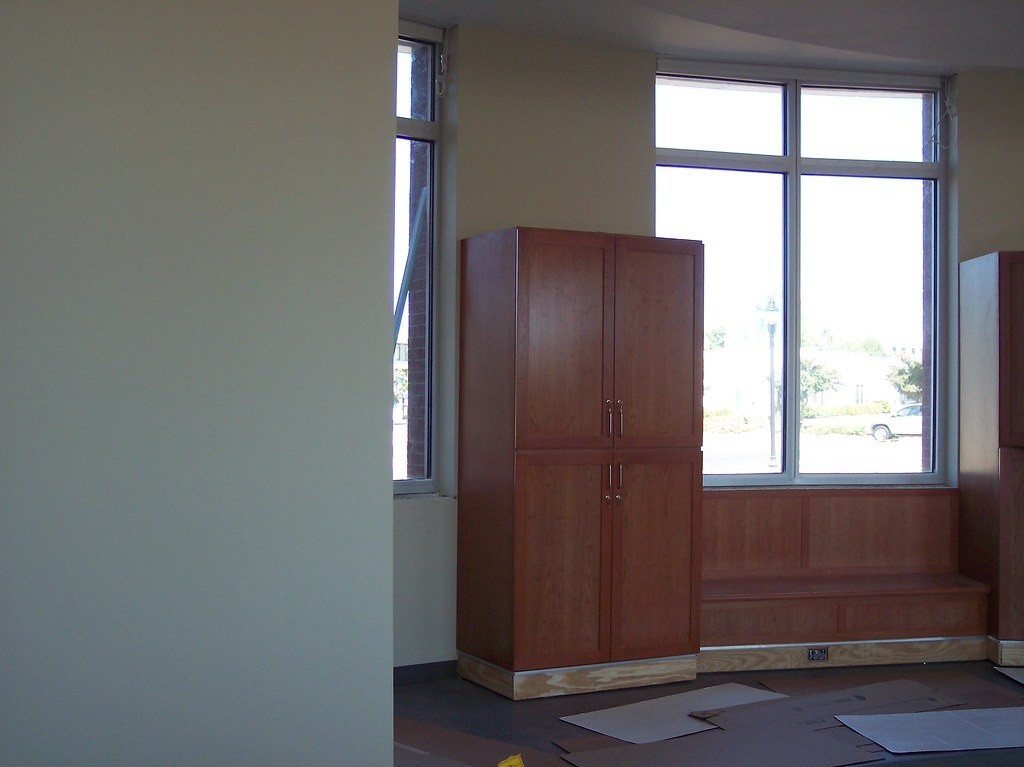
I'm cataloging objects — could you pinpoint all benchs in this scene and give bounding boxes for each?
[702,489,996,648]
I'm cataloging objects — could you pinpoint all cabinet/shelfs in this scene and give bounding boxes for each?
[456,225,706,673]
[958,251,1023,644]
